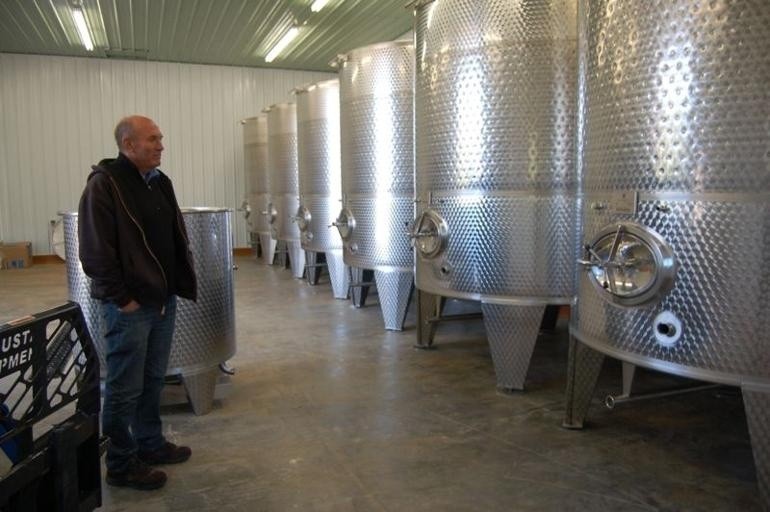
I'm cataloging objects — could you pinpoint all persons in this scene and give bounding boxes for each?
[78,115,198,490]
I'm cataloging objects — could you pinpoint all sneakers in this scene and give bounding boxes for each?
[105,441,192,490]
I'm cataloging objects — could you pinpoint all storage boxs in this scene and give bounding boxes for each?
[1,241,32,269]
